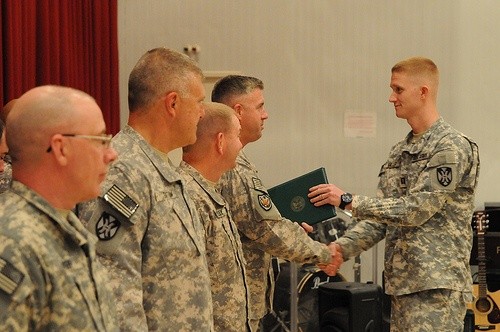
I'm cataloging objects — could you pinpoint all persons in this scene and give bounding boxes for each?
[211,74,343,332]
[0,99,22,193]
[308,57,479,332]
[178,100,252,332]
[85,48,214,332]
[2,83,119,332]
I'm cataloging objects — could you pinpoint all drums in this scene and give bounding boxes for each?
[263,261,347,332]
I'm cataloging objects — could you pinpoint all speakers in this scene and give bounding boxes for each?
[316,281,383,332]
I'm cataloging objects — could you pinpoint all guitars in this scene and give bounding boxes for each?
[466,211,500,332]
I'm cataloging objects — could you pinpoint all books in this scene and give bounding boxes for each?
[265,167,336,227]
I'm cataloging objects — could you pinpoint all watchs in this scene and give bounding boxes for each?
[338,192,353,209]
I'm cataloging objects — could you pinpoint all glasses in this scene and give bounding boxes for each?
[46,133,112,153]
[0,151,12,164]
